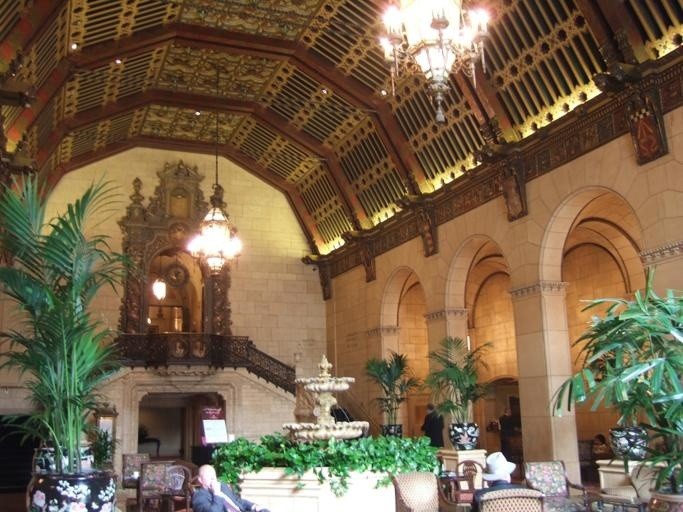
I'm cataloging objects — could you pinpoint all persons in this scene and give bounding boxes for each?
[420,404,444,448]
[498,408,522,461]
[591,433,615,482]
[471,452,524,512]
[192,464,271,512]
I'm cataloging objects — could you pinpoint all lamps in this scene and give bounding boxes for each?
[151,256,167,303]
[185,62,244,278]
[375,0,496,124]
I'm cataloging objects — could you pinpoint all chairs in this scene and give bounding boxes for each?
[385,442,647,512]
[120,452,203,512]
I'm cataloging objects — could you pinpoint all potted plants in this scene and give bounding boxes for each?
[358,347,424,440]
[579,343,651,461]
[0,168,145,512]
[412,334,498,451]
[544,261,683,512]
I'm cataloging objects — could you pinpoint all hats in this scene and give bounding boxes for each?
[482,451,517,482]
